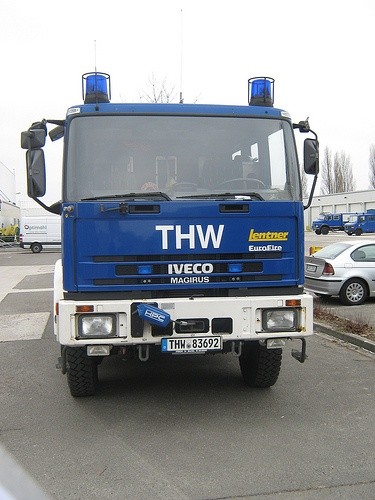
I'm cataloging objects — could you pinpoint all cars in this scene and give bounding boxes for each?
[304,239,375,306]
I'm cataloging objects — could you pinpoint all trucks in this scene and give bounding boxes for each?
[343,208,375,236]
[19,71,320,398]
[312,211,367,235]
[20,216,61,254]
